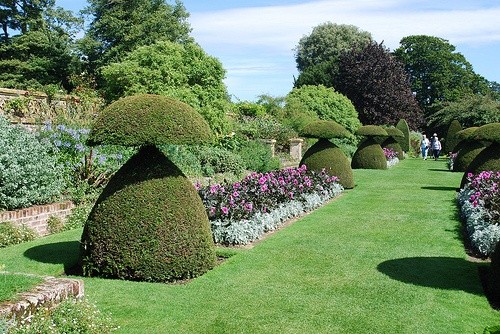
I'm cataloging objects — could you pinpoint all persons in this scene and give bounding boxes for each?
[419,135,429,161]
[430,133,437,159]
[432,138,442,160]
